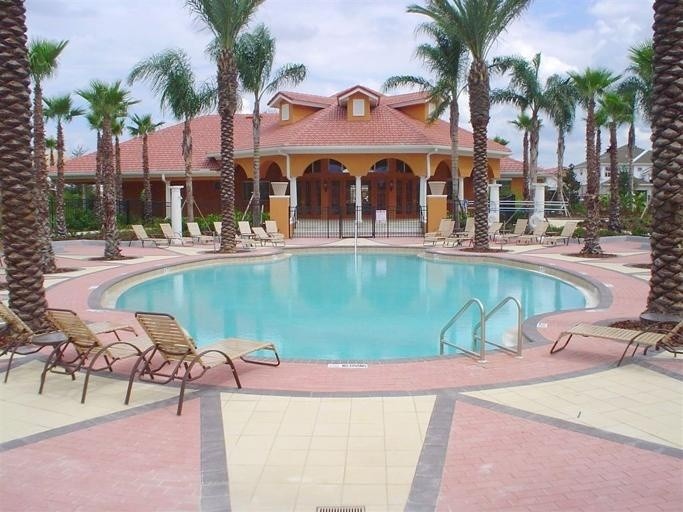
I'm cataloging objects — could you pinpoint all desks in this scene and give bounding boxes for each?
[640,313,683,357]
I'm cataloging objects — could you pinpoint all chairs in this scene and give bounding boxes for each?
[0,301,196,403]
[550,319,683,365]
[124,310,281,416]
[422,216,581,248]
[128,219,287,248]
